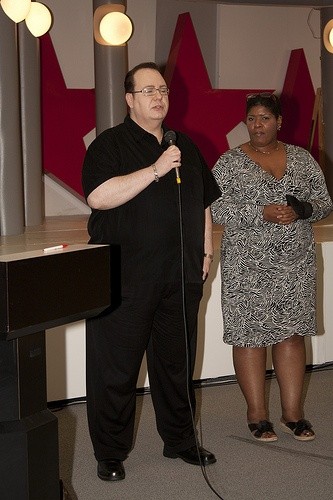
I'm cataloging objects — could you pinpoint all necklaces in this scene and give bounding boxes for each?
[249,141,277,154]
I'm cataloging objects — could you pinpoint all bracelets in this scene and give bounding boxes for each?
[204,253,214,262]
[151,164,159,182]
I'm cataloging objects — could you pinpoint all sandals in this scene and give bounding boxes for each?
[246,419,279,442]
[280,416,316,441]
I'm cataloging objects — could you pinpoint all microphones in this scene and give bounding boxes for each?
[165,130,182,184]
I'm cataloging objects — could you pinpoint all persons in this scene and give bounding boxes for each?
[82,62,223,480]
[209,92,333,441]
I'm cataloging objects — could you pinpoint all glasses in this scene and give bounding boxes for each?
[131,86,169,97]
[246,92,273,103]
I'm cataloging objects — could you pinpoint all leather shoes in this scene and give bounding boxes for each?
[163,443,216,465]
[96,458,126,481]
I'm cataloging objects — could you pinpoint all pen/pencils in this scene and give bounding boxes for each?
[42,244,69,252]
